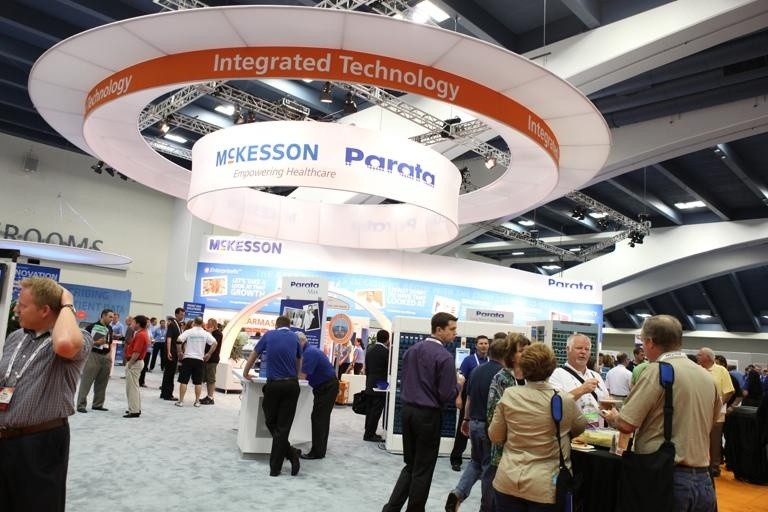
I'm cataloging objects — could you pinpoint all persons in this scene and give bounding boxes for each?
[76,306,223,418]
[242,316,303,477]
[1,278,94,512]
[293,331,339,460]
[742,363,768,382]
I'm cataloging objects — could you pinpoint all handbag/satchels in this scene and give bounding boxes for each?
[352,389,367,415]
[616,362,676,512]
[551,390,573,512]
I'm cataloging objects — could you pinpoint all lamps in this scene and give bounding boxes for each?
[320,81,334,104]
[343,90,357,114]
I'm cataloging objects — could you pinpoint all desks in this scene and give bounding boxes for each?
[725,404,758,485]
[232,368,314,458]
[571,445,624,512]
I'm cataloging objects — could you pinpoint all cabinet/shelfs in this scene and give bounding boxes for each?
[529,321,598,375]
[384,317,531,458]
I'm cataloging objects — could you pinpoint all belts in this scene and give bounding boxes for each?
[0,419,63,438]
[267,376,296,382]
[671,464,709,473]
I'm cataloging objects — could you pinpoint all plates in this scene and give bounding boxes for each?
[567,444,595,453]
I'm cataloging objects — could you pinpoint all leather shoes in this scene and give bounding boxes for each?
[164,397,178,401]
[123,414,139,417]
[140,384,147,387]
[160,395,173,398]
[92,406,108,411]
[451,462,460,471]
[126,410,141,414]
[363,434,385,442]
[289,449,301,475]
[301,450,321,459]
[375,433,381,438]
[270,470,280,476]
[77,409,87,413]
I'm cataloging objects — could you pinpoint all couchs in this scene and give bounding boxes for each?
[340,374,367,406]
[215,362,244,394]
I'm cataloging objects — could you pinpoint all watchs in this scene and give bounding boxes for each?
[61,303,78,314]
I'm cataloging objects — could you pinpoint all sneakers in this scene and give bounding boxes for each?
[713,467,720,476]
[200,397,214,404]
[445,489,461,512]
[175,401,183,407]
[193,401,200,407]
